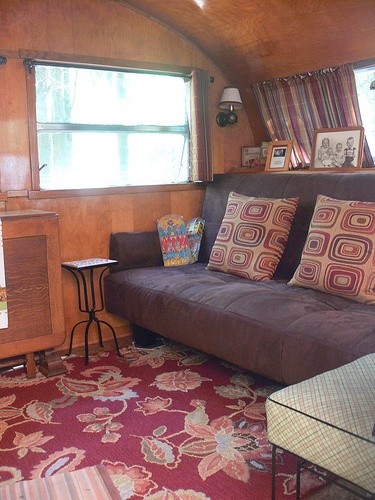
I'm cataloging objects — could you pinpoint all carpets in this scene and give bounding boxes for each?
[0,464,122,500]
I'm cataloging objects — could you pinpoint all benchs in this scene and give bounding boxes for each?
[265,352,375,500]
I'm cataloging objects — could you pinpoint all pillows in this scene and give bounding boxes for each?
[288,194,375,305]
[207,192,299,282]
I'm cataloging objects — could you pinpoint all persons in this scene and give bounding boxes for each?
[316,136,357,169]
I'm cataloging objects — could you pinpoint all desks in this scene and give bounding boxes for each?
[0,209,66,380]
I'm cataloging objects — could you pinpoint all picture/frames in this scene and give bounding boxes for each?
[310,126,364,171]
[264,140,292,172]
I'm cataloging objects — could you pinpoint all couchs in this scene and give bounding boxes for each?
[103,173,375,386]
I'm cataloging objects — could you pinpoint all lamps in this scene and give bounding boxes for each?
[216,88,243,127]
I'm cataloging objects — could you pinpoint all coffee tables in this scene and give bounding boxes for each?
[62,258,125,366]
